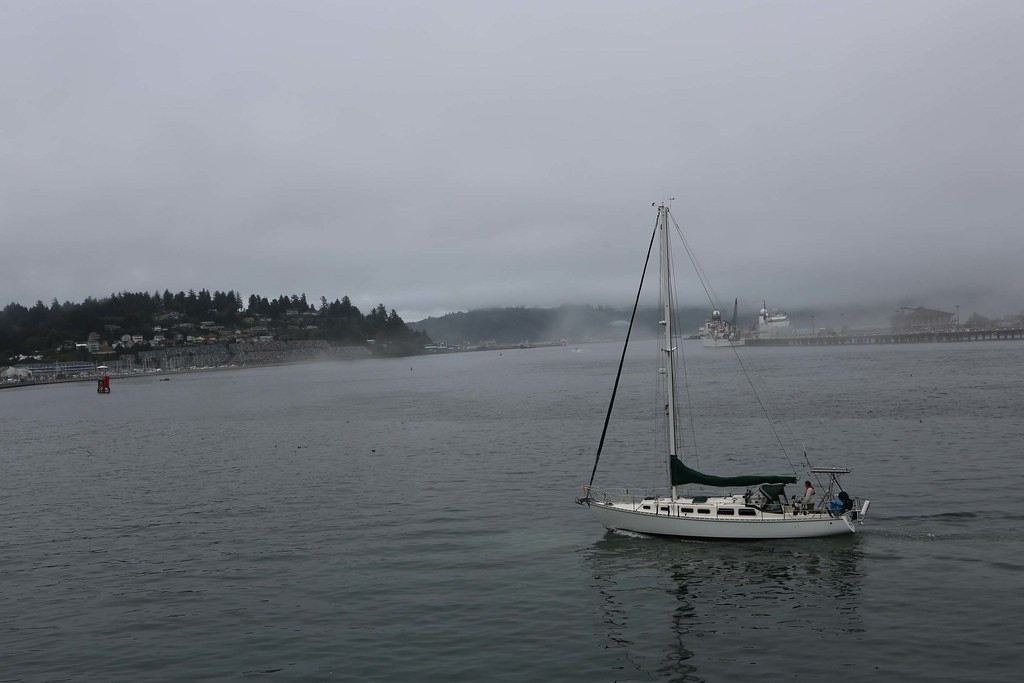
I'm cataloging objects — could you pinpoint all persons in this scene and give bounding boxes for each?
[803,480,816,511]
[838,491,858,521]
[97,371,111,394]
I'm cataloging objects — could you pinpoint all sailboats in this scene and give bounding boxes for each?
[572,198,870,540]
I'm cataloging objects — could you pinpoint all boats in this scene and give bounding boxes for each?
[699,297,746,346]
[749,300,796,338]
[422,335,567,354]
